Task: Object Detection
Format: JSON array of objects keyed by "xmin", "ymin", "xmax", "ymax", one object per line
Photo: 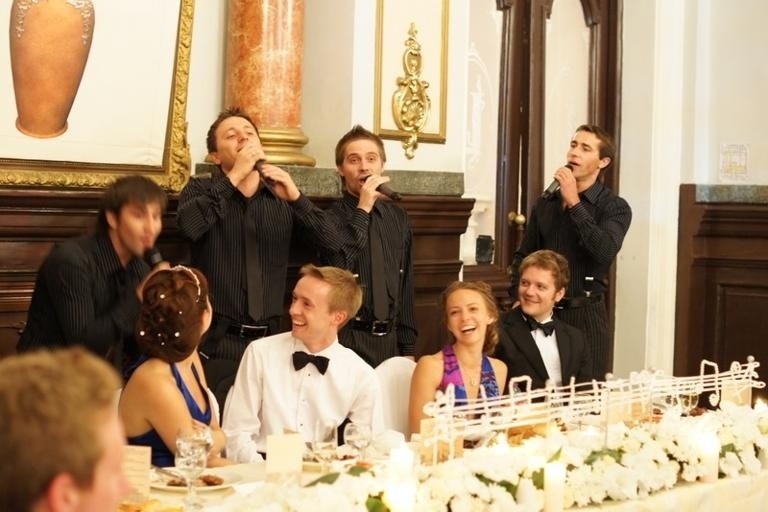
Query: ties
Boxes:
[
  {"xmin": 241, "ymin": 197, "xmax": 267, "ymax": 321},
  {"xmin": 367, "ymin": 212, "xmax": 391, "ymax": 321}
]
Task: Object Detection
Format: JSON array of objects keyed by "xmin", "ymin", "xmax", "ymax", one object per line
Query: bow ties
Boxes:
[
  {"xmin": 293, "ymin": 351, "xmax": 330, "ymax": 374},
  {"xmin": 527, "ymin": 319, "xmax": 556, "ymax": 337}
]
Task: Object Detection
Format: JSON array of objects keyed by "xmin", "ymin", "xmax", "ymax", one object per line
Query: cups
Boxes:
[{"xmin": 263, "ymin": 432, "xmax": 298, "ymax": 486}]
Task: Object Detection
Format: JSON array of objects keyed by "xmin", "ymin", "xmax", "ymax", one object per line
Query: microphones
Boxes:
[
  {"xmin": 255, "ymin": 158, "xmax": 276, "ymax": 188},
  {"xmin": 365, "ymin": 174, "xmax": 402, "ymax": 201},
  {"xmin": 142, "ymin": 246, "xmax": 162, "ymax": 270},
  {"xmin": 543, "ymin": 164, "xmax": 573, "ymax": 199}
]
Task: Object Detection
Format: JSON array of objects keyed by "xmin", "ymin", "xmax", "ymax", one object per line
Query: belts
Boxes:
[
  {"xmin": 553, "ymin": 296, "xmax": 601, "ymax": 309},
  {"xmin": 349, "ymin": 320, "xmax": 391, "ymax": 336},
  {"xmin": 229, "ymin": 324, "xmax": 268, "ymax": 341}
]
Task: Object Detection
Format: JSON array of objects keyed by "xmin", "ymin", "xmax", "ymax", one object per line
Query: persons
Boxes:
[
  {"xmin": 223, "ymin": 263, "xmax": 388, "ymax": 464},
  {"xmin": 409, "ymin": 279, "xmax": 508, "ymax": 434},
  {"xmin": 0, "ymin": 346, "xmax": 134, "ymax": 512},
  {"xmin": 16, "ymin": 175, "xmax": 163, "ymax": 361},
  {"xmin": 174, "ymin": 104, "xmax": 343, "ymax": 412},
  {"xmin": 322, "ymin": 124, "xmax": 418, "ymax": 368},
  {"xmin": 118, "ymin": 264, "xmax": 235, "ymax": 468},
  {"xmin": 513, "ymin": 124, "xmax": 631, "ymax": 383},
  {"xmin": 493, "ymin": 250, "xmax": 596, "ymax": 403}
]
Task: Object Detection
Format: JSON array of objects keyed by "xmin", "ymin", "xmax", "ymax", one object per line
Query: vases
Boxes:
[{"xmin": 9, "ymin": 0, "xmax": 95, "ymax": 139}]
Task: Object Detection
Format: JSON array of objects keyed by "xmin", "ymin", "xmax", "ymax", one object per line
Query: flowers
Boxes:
[{"xmin": 279, "ymin": 401, "xmax": 767, "ymax": 512}]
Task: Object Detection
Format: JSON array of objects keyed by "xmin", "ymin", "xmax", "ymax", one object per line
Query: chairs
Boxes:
[{"xmin": 368, "ymin": 354, "xmax": 417, "ymax": 442}]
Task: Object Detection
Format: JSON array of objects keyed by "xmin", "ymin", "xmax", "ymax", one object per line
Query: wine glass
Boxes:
[
  {"xmin": 311, "ymin": 417, "xmax": 339, "ymax": 477},
  {"xmin": 344, "ymin": 422, "xmax": 375, "ymax": 464},
  {"xmin": 172, "ymin": 422, "xmax": 213, "ymax": 512}
]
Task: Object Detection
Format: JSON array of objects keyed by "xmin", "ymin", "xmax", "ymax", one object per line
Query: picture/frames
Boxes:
[{"xmin": 0, "ymin": 0, "xmax": 194, "ymax": 199}]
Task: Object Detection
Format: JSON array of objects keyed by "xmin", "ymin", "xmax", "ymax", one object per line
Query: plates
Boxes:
[
  {"xmin": 299, "ymin": 444, "xmax": 356, "ymax": 472},
  {"xmin": 150, "ymin": 465, "xmax": 242, "ymax": 492}
]
